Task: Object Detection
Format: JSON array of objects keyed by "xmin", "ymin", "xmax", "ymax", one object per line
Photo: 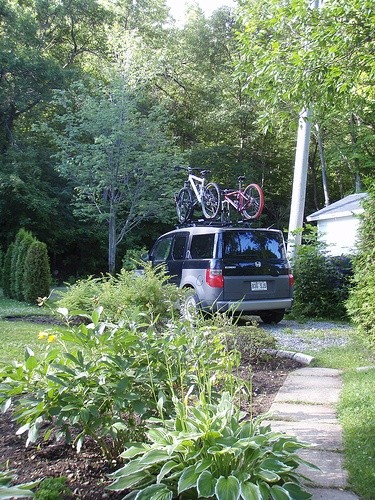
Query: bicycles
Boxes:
[{"xmin": 174, "ymin": 166, "xmax": 264, "ymax": 224}]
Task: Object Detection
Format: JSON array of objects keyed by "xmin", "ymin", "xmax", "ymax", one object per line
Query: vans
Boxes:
[{"xmin": 142, "ymin": 227, "xmax": 294, "ymax": 323}]
